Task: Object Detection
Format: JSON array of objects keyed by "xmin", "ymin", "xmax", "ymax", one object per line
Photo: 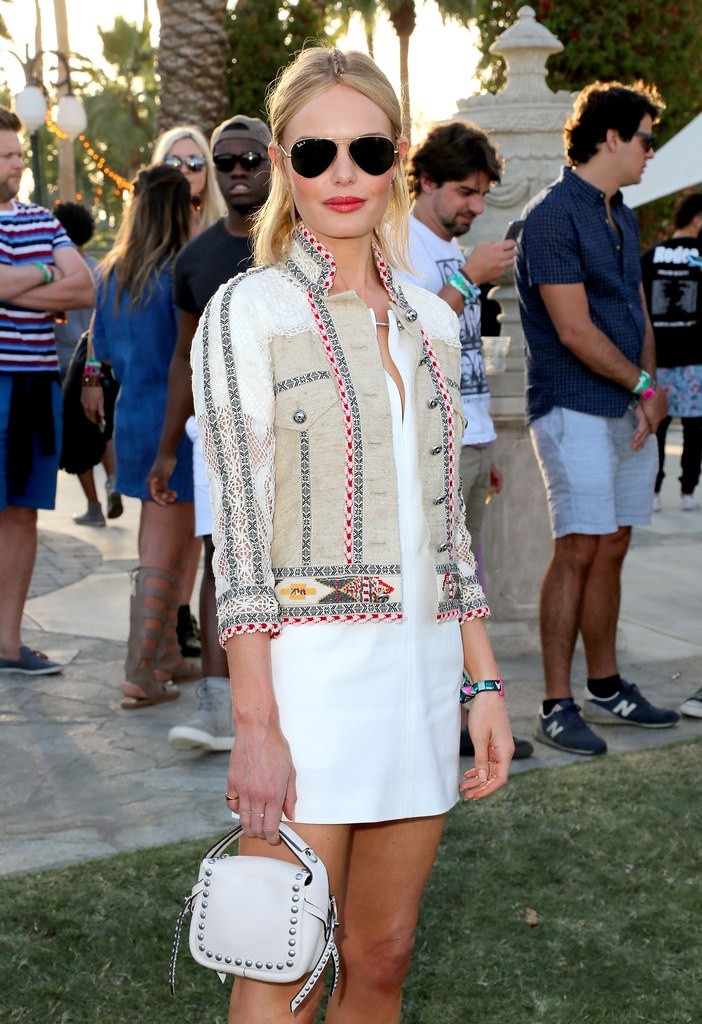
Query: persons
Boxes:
[
  {"xmin": 384, "ymin": 121, "xmax": 534, "ymax": 758},
  {"xmin": 0, "ymin": 109, "xmax": 97, "ymax": 675},
  {"xmin": 189, "ymin": 48, "xmax": 515, "ymax": 1024},
  {"xmin": 91, "ymin": 163, "xmax": 202, "ymax": 708},
  {"xmin": 640, "ymin": 192, "xmax": 702, "ymax": 511},
  {"xmin": 52, "ymin": 202, "xmax": 124, "ymax": 527},
  {"xmin": 81, "ymin": 127, "xmax": 229, "ymax": 657},
  {"xmin": 513, "ymin": 82, "xmax": 681, "ymax": 754},
  {"xmin": 147, "ymin": 115, "xmax": 272, "ymax": 751}
]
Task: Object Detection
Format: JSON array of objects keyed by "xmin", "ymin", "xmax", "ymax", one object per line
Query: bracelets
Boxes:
[
  {"xmin": 33, "ymin": 262, "xmax": 54, "ymax": 282},
  {"xmin": 628, "ymin": 395, "xmax": 639, "ymax": 410},
  {"xmin": 447, "ymin": 269, "xmax": 481, "ymax": 305},
  {"xmin": 460, "ymin": 674, "xmax": 504, "ymax": 704},
  {"xmin": 632, "ymin": 370, "xmax": 656, "ymax": 400},
  {"xmin": 82, "ymin": 358, "xmax": 104, "ymax": 387}
]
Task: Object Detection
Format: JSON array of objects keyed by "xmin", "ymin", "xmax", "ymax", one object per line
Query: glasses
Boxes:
[
  {"xmin": 213, "ymin": 152, "xmax": 267, "ymax": 172},
  {"xmin": 278, "ymin": 136, "xmax": 399, "ymax": 176},
  {"xmin": 636, "ymin": 132, "xmax": 656, "ymax": 152},
  {"xmin": 165, "ymin": 155, "xmax": 206, "ymax": 171}
]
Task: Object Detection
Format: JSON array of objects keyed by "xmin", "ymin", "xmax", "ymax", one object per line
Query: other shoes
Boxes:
[
  {"xmin": 73, "ymin": 503, "xmax": 105, "ymax": 526},
  {"xmin": 652, "ymin": 495, "xmax": 661, "ymax": 512},
  {"xmin": 105, "ymin": 475, "xmax": 124, "ymax": 518},
  {"xmin": 0, "ymin": 646, "xmax": 64, "ymax": 674},
  {"xmin": 682, "ymin": 494, "xmax": 693, "ymax": 511},
  {"xmin": 462, "ymin": 727, "xmax": 533, "ymax": 759},
  {"xmin": 680, "ymin": 687, "xmax": 702, "ymax": 718}
]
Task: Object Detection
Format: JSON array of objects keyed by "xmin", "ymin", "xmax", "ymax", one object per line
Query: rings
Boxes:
[
  {"xmin": 248, "ymin": 810, "xmax": 264, "ymax": 818},
  {"xmin": 225, "ymin": 793, "xmax": 239, "ymax": 800}
]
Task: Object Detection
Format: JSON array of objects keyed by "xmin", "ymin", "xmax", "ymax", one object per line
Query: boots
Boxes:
[
  {"xmin": 154, "ymin": 561, "xmax": 202, "ymax": 681},
  {"xmin": 177, "ymin": 604, "xmax": 204, "ymax": 671},
  {"xmin": 166, "ymin": 677, "xmax": 233, "ymax": 753},
  {"xmin": 120, "ymin": 566, "xmax": 180, "ymax": 708}
]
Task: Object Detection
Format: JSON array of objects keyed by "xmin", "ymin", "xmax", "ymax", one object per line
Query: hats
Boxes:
[{"xmin": 210, "ymin": 114, "xmax": 271, "ymax": 154}]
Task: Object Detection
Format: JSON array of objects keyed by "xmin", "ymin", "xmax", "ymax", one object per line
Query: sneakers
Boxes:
[
  {"xmin": 583, "ymin": 681, "xmax": 679, "ymax": 728},
  {"xmin": 534, "ymin": 700, "xmax": 606, "ymax": 753}
]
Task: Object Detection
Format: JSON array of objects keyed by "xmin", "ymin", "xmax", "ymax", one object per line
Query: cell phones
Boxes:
[
  {"xmin": 503, "ymin": 221, "xmax": 525, "ymax": 241},
  {"xmin": 96, "ymin": 411, "xmax": 106, "ymax": 433}
]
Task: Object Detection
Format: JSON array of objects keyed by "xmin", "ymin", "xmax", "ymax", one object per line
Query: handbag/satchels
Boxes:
[{"xmin": 170, "ymin": 821, "xmax": 340, "ymax": 1011}]
